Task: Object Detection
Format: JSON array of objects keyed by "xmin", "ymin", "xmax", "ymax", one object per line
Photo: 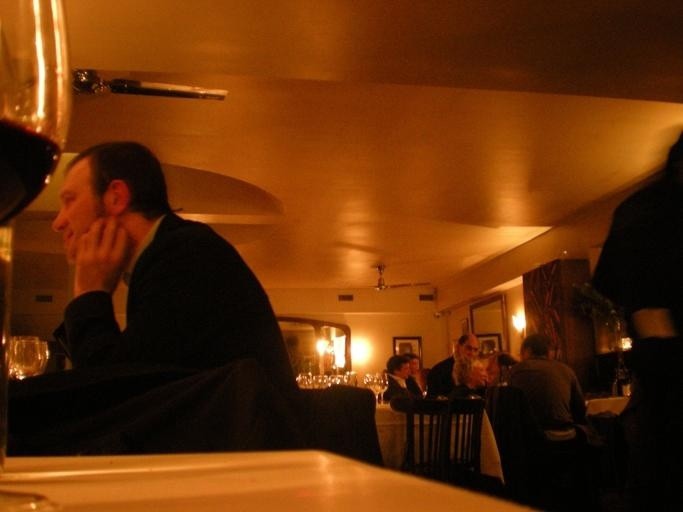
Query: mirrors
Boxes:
[{"xmin": 274, "ymin": 317, "xmax": 352, "ymax": 377}]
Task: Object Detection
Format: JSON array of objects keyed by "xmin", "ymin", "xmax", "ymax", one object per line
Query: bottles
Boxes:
[{"xmin": 609, "ymin": 360, "xmax": 631, "ymax": 396}]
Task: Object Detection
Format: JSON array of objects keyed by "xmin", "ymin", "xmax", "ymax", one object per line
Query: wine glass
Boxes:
[{"xmin": 295, "ymin": 371, "xmax": 387, "ymax": 407}]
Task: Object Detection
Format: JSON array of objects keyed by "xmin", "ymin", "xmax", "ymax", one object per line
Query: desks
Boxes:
[{"xmin": 0, "ymin": 449, "xmax": 545, "ymax": 512}]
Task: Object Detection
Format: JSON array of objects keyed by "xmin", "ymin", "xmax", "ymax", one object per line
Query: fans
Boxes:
[{"xmin": 334, "ymin": 260, "xmax": 431, "ymax": 292}]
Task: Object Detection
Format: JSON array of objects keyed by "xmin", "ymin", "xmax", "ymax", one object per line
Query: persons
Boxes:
[
  {"xmin": 585, "ymin": 128, "xmax": 683, "ymax": 509},
  {"xmin": 504, "ymin": 333, "xmax": 593, "ymax": 447},
  {"xmin": 402, "ymin": 351, "xmax": 425, "ymax": 400},
  {"xmin": 478, "ymin": 338, "xmax": 496, "ymax": 360},
  {"xmin": 0, "ymin": 137, "xmax": 317, "ymax": 455},
  {"xmin": 490, "ymin": 353, "xmax": 517, "ymax": 385},
  {"xmin": 445, "ymin": 359, "xmax": 487, "ymax": 402},
  {"xmin": 377, "ymin": 355, "xmax": 416, "ymax": 405},
  {"xmin": 425, "ymin": 332, "xmax": 488, "ymax": 397}
]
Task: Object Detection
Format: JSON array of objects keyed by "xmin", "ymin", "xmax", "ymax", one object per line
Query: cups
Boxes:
[
  {"xmin": 0, "ymin": 0, "xmax": 69, "ymax": 225},
  {"xmin": 4, "ymin": 335, "xmax": 49, "ymax": 380}
]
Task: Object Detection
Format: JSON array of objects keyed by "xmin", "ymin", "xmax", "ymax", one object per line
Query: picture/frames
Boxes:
[
  {"xmin": 392, "ymin": 336, "xmax": 422, "ymax": 359},
  {"xmin": 474, "ymin": 333, "xmax": 501, "ymax": 359},
  {"xmin": 467, "ymin": 293, "xmax": 510, "ymax": 355}
]
